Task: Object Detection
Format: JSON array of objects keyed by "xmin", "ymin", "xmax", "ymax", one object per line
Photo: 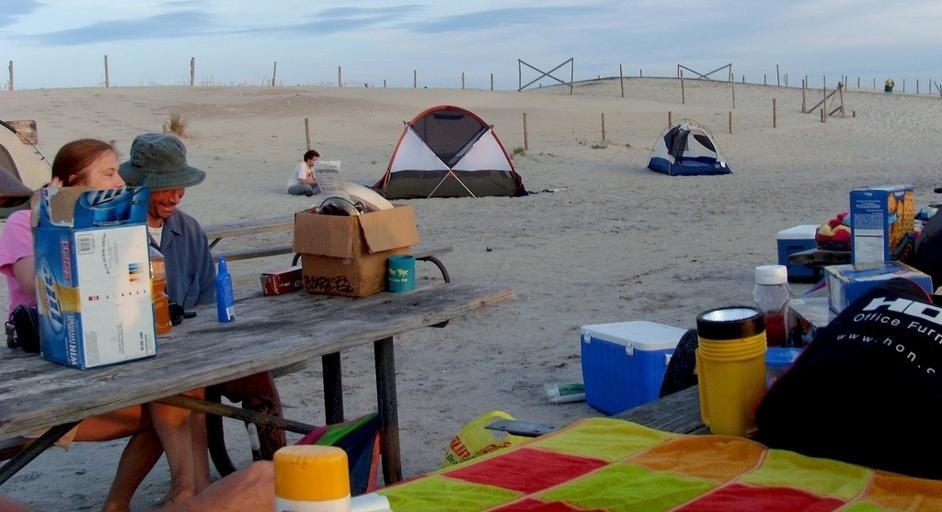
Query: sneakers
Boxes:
[{"xmin": 301, "ymin": 191, "xmax": 313, "ymax": 196}]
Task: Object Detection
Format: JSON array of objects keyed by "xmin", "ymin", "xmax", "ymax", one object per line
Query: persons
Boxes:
[
  {"xmin": 287, "ymin": 148, "xmax": 322, "ymax": 196},
  {"xmin": 0, "ymin": 132, "xmax": 288, "ymax": 512}
]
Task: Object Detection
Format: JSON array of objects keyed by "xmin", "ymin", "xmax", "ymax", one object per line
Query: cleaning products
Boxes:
[{"xmin": 217, "ymin": 256, "xmax": 236, "ymax": 322}]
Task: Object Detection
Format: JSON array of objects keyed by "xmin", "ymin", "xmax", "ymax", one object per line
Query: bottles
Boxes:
[
  {"xmin": 753, "ymin": 265, "xmax": 792, "ymax": 347},
  {"xmin": 214, "ymin": 253, "xmax": 234, "ymax": 321},
  {"xmin": 272, "ymin": 444, "xmax": 353, "ymax": 512}
]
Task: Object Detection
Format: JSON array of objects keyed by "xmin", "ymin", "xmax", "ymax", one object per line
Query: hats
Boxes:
[{"xmin": 118, "ymin": 133, "xmax": 206, "ymax": 191}]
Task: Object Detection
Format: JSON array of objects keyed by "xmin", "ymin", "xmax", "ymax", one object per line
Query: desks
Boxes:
[
  {"xmin": 360, "ymin": 365, "xmax": 942, "ymax": 512},
  {"xmin": 0, "ymin": 268, "xmax": 513, "ymax": 512}
]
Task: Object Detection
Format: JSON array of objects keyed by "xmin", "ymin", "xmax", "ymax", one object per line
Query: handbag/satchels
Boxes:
[{"xmin": 13, "ymin": 303, "xmax": 40, "ymax": 353}]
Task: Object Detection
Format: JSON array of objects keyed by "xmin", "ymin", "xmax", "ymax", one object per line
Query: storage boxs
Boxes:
[
  {"xmin": 292, "ymin": 201, "xmax": 420, "ymax": 299},
  {"xmin": 849, "ymin": 183, "xmax": 914, "ymax": 265},
  {"xmin": 581, "ymin": 320, "xmax": 688, "ymax": 418},
  {"xmin": 823, "ymin": 260, "xmax": 933, "ymax": 316},
  {"xmin": 777, "ymin": 223, "xmax": 828, "ymax": 284},
  {"xmin": 27, "ymin": 185, "xmax": 157, "ymax": 369}
]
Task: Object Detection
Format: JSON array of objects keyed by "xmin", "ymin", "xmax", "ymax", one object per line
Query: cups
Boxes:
[{"xmin": 386, "ymin": 254, "xmax": 414, "ymax": 294}]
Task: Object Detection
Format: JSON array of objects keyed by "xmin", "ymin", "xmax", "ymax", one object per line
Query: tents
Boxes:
[
  {"xmin": 375, "ymin": 105, "xmax": 528, "ymax": 201},
  {"xmin": 647, "ymin": 121, "xmax": 733, "ymax": 177},
  {"xmin": 0, "ymin": 119, "xmax": 57, "ymax": 215}
]
